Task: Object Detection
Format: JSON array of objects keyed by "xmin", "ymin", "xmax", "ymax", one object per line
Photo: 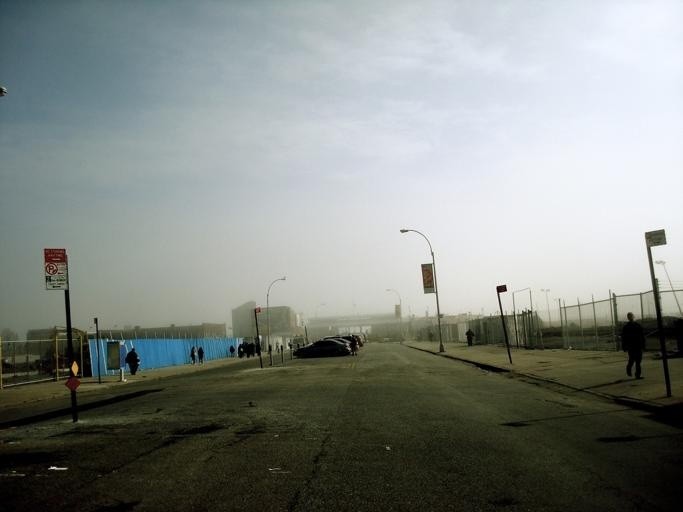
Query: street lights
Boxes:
[
  {"xmin": 540, "ymin": 288, "xmax": 552, "ymax": 328},
  {"xmin": 400, "ymin": 229, "xmax": 445, "ymax": 352},
  {"xmin": 385, "ymin": 288, "xmax": 403, "ymax": 345},
  {"xmin": 267, "ymin": 276, "xmax": 286, "ymax": 366},
  {"xmin": 655, "ymin": 260, "xmax": 682, "ymax": 316}
]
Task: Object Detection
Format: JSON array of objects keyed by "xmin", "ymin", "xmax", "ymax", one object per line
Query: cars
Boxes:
[{"xmin": 293, "ymin": 333, "xmax": 369, "ymax": 358}]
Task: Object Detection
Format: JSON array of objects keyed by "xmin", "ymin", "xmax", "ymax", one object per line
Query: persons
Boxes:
[
  {"xmin": 198, "ymin": 347, "xmax": 204, "ymax": 362},
  {"xmin": 191, "ymin": 347, "xmax": 197, "ymax": 363},
  {"xmin": 126, "ymin": 348, "xmax": 139, "ymax": 375},
  {"xmin": 621, "ymin": 312, "xmax": 647, "ymax": 378},
  {"xmin": 465, "ymin": 329, "xmax": 474, "ymax": 346},
  {"xmin": 237, "ymin": 343, "xmax": 260, "ymax": 359},
  {"xmin": 351, "ymin": 335, "xmax": 358, "ymax": 356},
  {"xmin": 230, "ymin": 346, "xmax": 235, "ymax": 358}
]
